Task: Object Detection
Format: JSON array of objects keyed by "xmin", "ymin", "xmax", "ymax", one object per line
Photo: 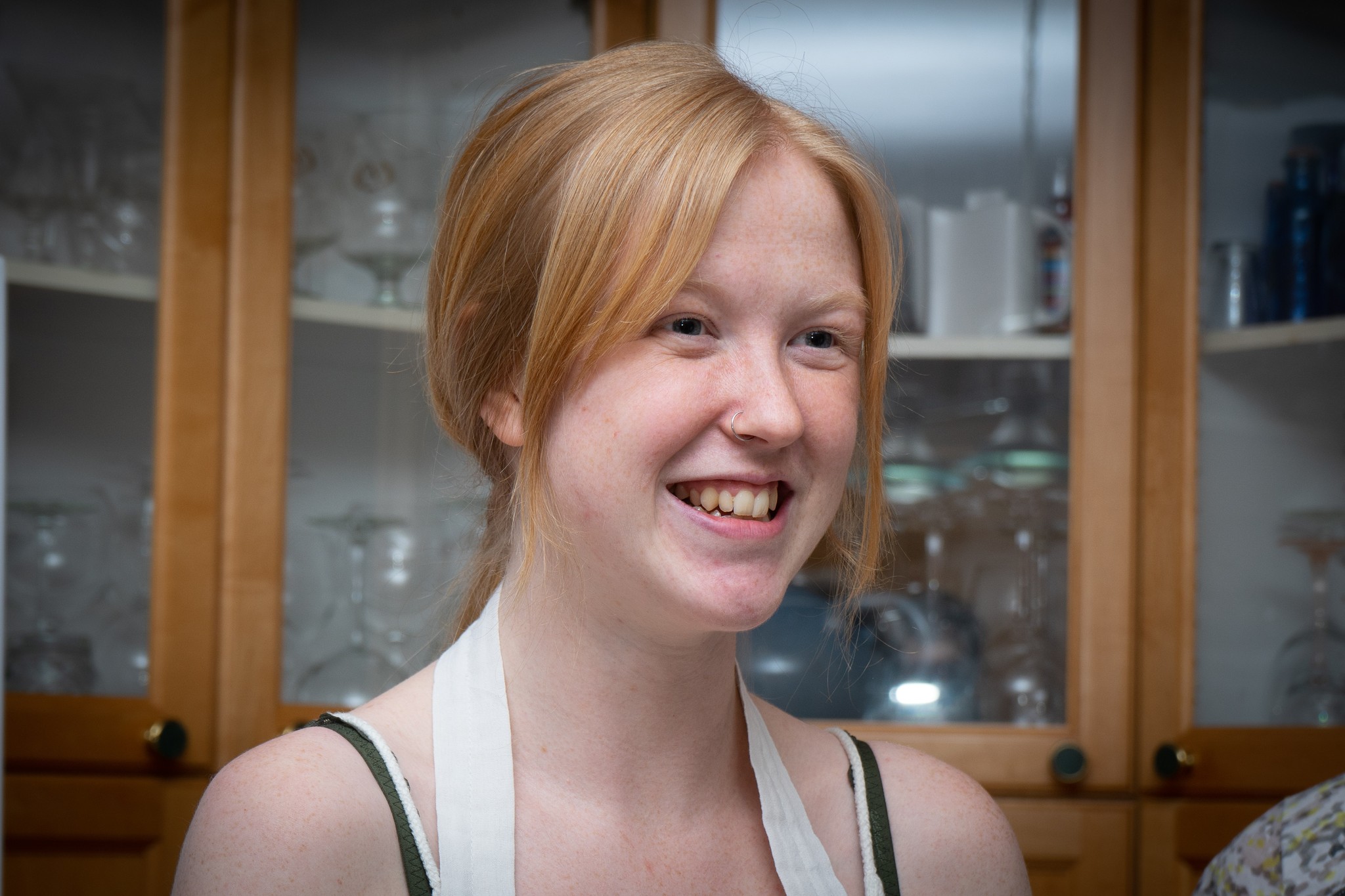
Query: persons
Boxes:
[{"xmin": 169, "ymin": 39, "xmax": 1032, "ymax": 896}]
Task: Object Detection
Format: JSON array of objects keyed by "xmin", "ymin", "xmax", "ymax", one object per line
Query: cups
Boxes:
[
  {"xmin": 928, "ymin": 206, "xmax": 1073, "ymax": 341},
  {"xmin": 1208, "ymin": 244, "xmax": 1259, "ymax": 329}
]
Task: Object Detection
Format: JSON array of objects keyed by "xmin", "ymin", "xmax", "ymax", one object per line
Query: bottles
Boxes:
[
  {"xmin": 845, "ymin": 369, "xmax": 1064, "ymax": 735},
  {"xmin": 1257, "ymin": 156, "xmax": 1328, "ymax": 323}
]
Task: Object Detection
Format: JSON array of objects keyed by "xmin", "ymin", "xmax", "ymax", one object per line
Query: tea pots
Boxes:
[{"xmin": 739, "ymin": 570, "xmax": 937, "ymax": 718}]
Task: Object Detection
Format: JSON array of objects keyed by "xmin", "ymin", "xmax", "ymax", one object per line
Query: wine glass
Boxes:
[
  {"xmin": 1279, "ymin": 511, "xmax": 1344, "ymax": 725},
  {"xmin": 3, "ymin": 496, "xmax": 152, "ymax": 700},
  {"xmin": 0, "ymin": 121, "xmax": 439, "ymax": 313},
  {"xmin": 287, "ymin": 507, "xmax": 487, "ymax": 707}
]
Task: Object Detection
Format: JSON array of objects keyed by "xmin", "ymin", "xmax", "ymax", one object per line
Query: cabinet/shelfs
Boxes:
[{"xmin": 1, "ymin": 1, "xmax": 1345, "ymax": 896}]
